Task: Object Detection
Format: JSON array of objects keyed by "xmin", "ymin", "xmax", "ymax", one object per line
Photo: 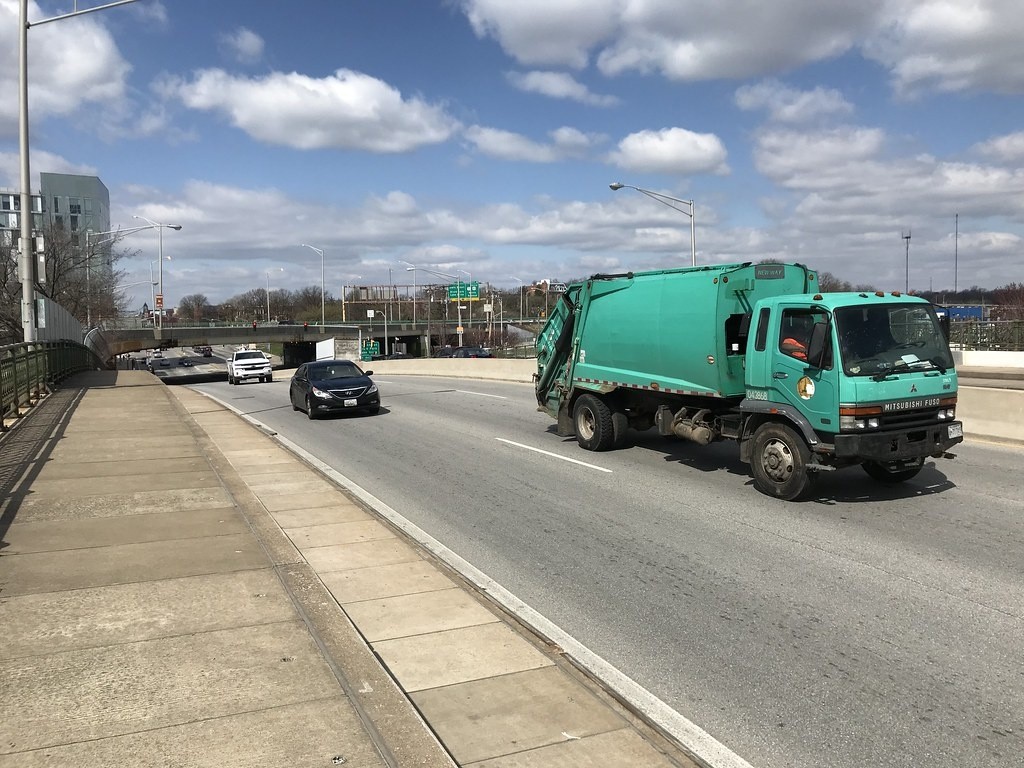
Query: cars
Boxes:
[
  {"xmin": 192, "ymin": 345, "xmax": 213, "ymax": 358},
  {"xmin": 160, "ymin": 358, "xmax": 170, "ymax": 366},
  {"xmin": 153, "ymin": 347, "xmax": 167, "ymax": 358},
  {"xmin": 372, "ymin": 345, "xmax": 496, "ymax": 361},
  {"xmin": 179, "ymin": 357, "xmax": 193, "ymax": 367},
  {"xmin": 290, "ymin": 358, "xmax": 380, "ymax": 420}
]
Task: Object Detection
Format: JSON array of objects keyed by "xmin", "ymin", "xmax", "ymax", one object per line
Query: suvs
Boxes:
[{"xmin": 226, "ymin": 348, "xmax": 273, "ymax": 386}]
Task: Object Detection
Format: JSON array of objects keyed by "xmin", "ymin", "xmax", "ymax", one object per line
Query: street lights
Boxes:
[
  {"xmin": 267, "ymin": 267, "xmax": 284, "ymax": 323},
  {"xmin": 151, "ymin": 255, "xmax": 172, "ymax": 329},
  {"xmin": 457, "ymin": 269, "xmax": 471, "ymax": 322},
  {"xmin": 376, "ymin": 310, "xmax": 388, "ymax": 356},
  {"xmin": 302, "ymin": 244, "xmax": 325, "ymax": 325},
  {"xmin": 132, "ymin": 215, "xmax": 163, "ymax": 329},
  {"xmin": 87, "ymin": 225, "xmax": 182, "ymax": 338},
  {"xmin": 609, "ymin": 182, "xmax": 696, "ymax": 267},
  {"xmin": 511, "ymin": 276, "xmax": 523, "ymax": 321},
  {"xmin": 408, "ymin": 266, "xmax": 462, "ymax": 347}
]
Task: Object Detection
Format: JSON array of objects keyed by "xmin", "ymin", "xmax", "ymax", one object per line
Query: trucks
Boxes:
[{"xmin": 532, "ymin": 261, "xmax": 965, "ymax": 502}]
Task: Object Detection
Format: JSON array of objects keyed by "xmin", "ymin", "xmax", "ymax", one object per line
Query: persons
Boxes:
[
  {"xmin": 782, "ymin": 317, "xmax": 815, "ymax": 358},
  {"xmin": 855, "ymin": 309, "xmax": 906, "ymax": 357}
]
